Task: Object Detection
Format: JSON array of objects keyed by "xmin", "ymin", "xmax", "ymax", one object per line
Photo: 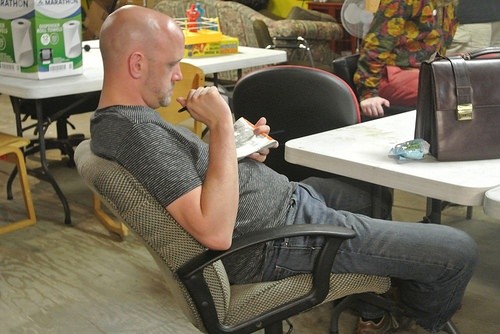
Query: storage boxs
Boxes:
[
  {"xmin": 0, "ymin": 0, "xmax": 83, "ymax": 80},
  {"xmin": 82, "ymin": 0, "xmax": 115, "ymax": 37}
]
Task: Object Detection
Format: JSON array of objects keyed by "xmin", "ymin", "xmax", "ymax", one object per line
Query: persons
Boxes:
[
  {"xmin": 353, "ymin": 0, "xmax": 465, "ymax": 118},
  {"xmin": 90, "ymin": 5, "xmax": 478, "ymax": 334}
]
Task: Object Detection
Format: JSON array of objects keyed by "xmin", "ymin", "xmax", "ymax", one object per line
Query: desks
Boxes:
[{"xmin": 0, "ymin": 40, "xmax": 500, "ymax": 225}]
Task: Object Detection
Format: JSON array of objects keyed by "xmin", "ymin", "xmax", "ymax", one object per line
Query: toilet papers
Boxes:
[
  {"xmin": 10, "ymin": 18, "xmax": 34, "ymax": 67},
  {"xmin": 62, "ymin": 20, "xmax": 82, "ymax": 59}
]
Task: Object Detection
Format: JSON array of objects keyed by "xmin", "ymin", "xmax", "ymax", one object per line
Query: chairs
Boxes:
[
  {"xmin": 92, "ymin": 62, "xmax": 204, "ymax": 238},
  {"xmin": 73, "ymin": 140, "xmax": 390, "ymax": 334},
  {"xmin": 252, "ymin": 19, "xmax": 315, "ymax": 69},
  {"xmin": 0, "ymin": 131, "xmax": 36, "ymax": 237},
  {"xmin": 232, "ymin": 65, "xmax": 360, "ymax": 182},
  {"xmin": 330, "ymin": 53, "xmax": 415, "ymax": 122}
]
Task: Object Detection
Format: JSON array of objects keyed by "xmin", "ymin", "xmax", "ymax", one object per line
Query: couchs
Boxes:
[{"xmin": 152, "ymin": 0, "xmax": 342, "ymax": 98}]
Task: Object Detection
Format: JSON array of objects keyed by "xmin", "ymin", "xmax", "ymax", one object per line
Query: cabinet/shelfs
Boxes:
[{"xmin": 307, "ymin": 2, "xmax": 357, "ymax": 54}]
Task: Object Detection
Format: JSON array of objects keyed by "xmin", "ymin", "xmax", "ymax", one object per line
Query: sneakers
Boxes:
[{"xmin": 356, "ymin": 316, "xmax": 387, "ymax": 334}]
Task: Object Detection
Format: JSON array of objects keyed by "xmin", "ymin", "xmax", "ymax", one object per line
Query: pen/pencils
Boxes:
[{"xmin": 178, "ymin": 106, "xmax": 187, "ymax": 113}]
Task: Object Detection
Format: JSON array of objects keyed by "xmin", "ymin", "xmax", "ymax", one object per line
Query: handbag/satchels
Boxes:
[{"xmin": 414, "ymin": 47, "xmax": 500, "ymax": 161}]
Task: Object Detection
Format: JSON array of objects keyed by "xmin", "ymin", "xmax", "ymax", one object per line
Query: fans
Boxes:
[{"xmin": 340, "ymin": 0, "xmax": 381, "ymax": 53}]
